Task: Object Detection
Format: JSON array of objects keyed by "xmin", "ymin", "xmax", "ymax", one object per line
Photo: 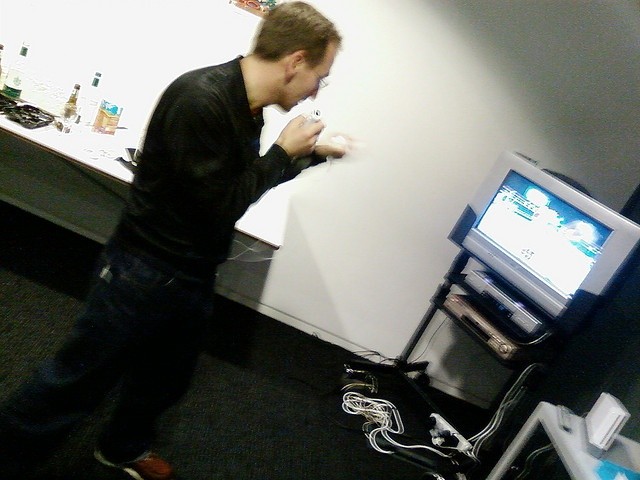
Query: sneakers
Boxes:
[{"xmin": 94, "ymin": 445, "xmax": 174, "ymax": 480}]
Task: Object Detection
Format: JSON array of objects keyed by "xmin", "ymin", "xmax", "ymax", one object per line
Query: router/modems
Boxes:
[{"xmin": 585, "ymin": 392, "xmax": 631, "ymax": 453}]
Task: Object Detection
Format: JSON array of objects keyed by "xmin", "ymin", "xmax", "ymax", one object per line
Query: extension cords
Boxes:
[{"xmin": 430, "ymin": 412, "xmax": 473, "ymax": 453}]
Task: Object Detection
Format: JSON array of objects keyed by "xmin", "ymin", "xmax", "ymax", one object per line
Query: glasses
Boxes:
[{"xmin": 310, "ymin": 67, "xmax": 331, "ymax": 90}]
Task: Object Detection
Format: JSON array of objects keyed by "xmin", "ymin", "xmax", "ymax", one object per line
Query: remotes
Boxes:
[{"xmin": 558, "ymin": 404, "xmax": 571, "ymax": 431}]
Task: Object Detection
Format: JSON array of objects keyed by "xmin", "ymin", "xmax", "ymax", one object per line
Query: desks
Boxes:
[{"xmin": 479, "ymin": 401, "xmax": 640, "ymax": 480}]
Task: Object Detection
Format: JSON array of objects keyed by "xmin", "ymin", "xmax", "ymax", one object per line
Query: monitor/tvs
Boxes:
[{"xmin": 447, "ymin": 150, "xmax": 640, "ymax": 318}]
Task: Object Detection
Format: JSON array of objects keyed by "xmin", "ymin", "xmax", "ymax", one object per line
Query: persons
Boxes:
[{"xmin": 0, "ymin": 0, "xmax": 347, "ymax": 480}]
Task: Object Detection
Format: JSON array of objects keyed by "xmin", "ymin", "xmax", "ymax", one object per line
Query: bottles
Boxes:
[
  {"xmin": 82, "ymin": 71, "xmax": 102, "ymax": 126},
  {"xmin": 73, "ymin": 70, "xmax": 103, "ymax": 124},
  {"xmin": 56, "ymin": 83, "xmax": 82, "ymax": 137},
  {"xmin": 0, "ymin": 40, "xmax": 31, "ymax": 112}
]
